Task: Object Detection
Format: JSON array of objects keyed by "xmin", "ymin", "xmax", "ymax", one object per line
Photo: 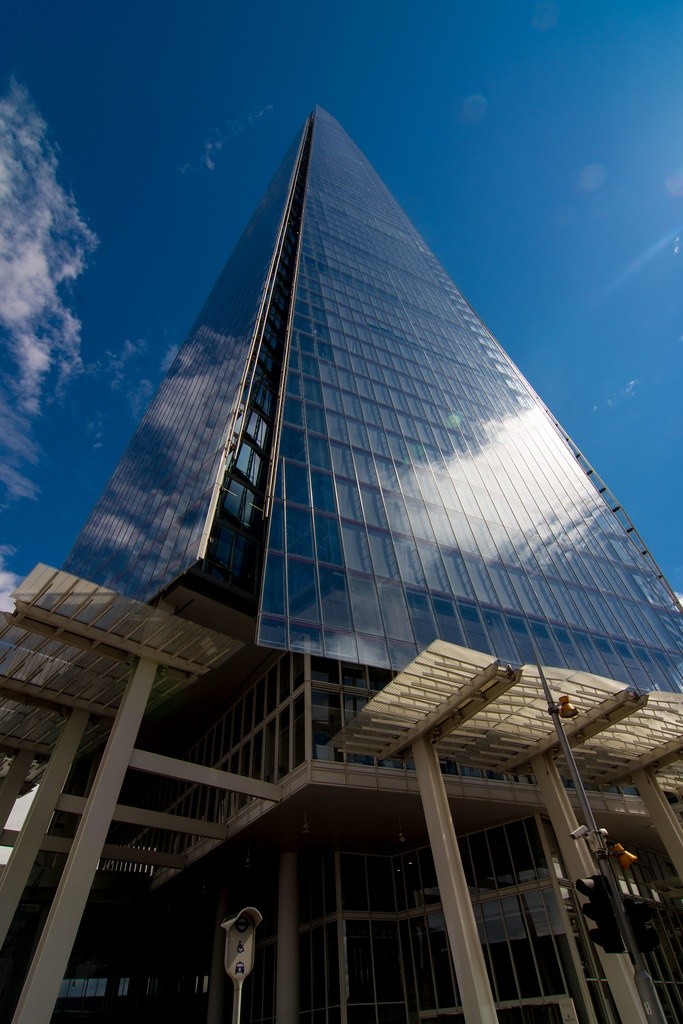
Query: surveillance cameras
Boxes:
[{"xmin": 570, "ymin": 825, "xmax": 588, "ymax": 840}]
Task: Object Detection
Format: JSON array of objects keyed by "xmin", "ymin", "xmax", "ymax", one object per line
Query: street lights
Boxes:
[{"xmin": 530, "ymin": 643, "xmax": 669, "ymax": 1024}]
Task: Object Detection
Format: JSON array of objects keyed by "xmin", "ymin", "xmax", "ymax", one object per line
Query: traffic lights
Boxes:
[{"xmin": 575, "ymin": 874, "xmax": 626, "ymax": 954}]
